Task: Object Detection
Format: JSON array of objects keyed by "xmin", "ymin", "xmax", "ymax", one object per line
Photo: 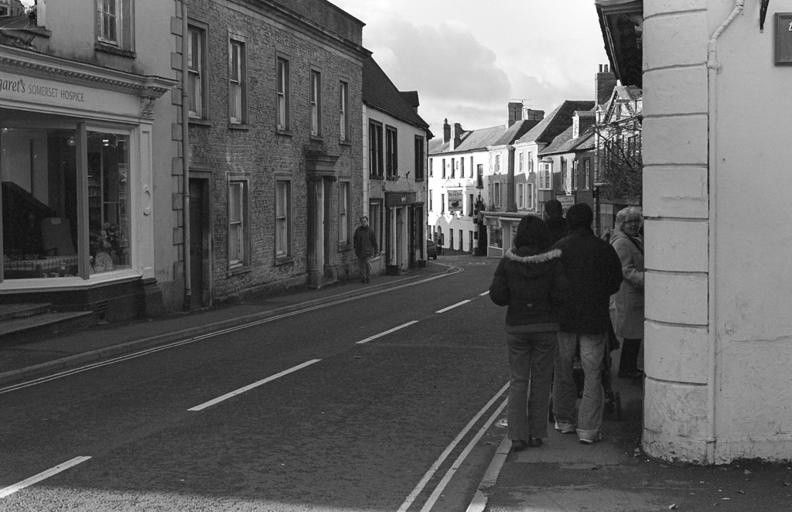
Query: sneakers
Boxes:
[
  {"xmin": 619, "ymin": 367, "xmax": 644, "ymax": 379},
  {"xmin": 510, "ymin": 434, "xmax": 542, "ymax": 452},
  {"xmin": 361, "ymin": 275, "xmax": 370, "ymax": 283},
  {"xmin": 553, "ymin": 419, "xmax": 606, "ymax": 444}
]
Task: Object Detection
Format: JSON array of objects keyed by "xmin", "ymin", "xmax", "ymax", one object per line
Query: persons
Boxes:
[
  {"xmin": 609, "ymin": 206, "xmax": 644, "ymax": 379},
  {"xmin": 489, "ymin": 199, "xmax": 623, "ymax": 450},
  {"xmin": 354, "ymin": 216, "xmax": 378, "ymax": 284}
]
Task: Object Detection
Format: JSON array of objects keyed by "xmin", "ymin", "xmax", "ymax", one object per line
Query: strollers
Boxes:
[{"xmin": 548, "ymin": 307, "xmax": 626, "ymax": 423}]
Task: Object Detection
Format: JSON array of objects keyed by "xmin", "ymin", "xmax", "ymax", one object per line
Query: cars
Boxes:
[{"xmin": 427, "ymin": 239, "xmax": 438, "ymax": 260}]
